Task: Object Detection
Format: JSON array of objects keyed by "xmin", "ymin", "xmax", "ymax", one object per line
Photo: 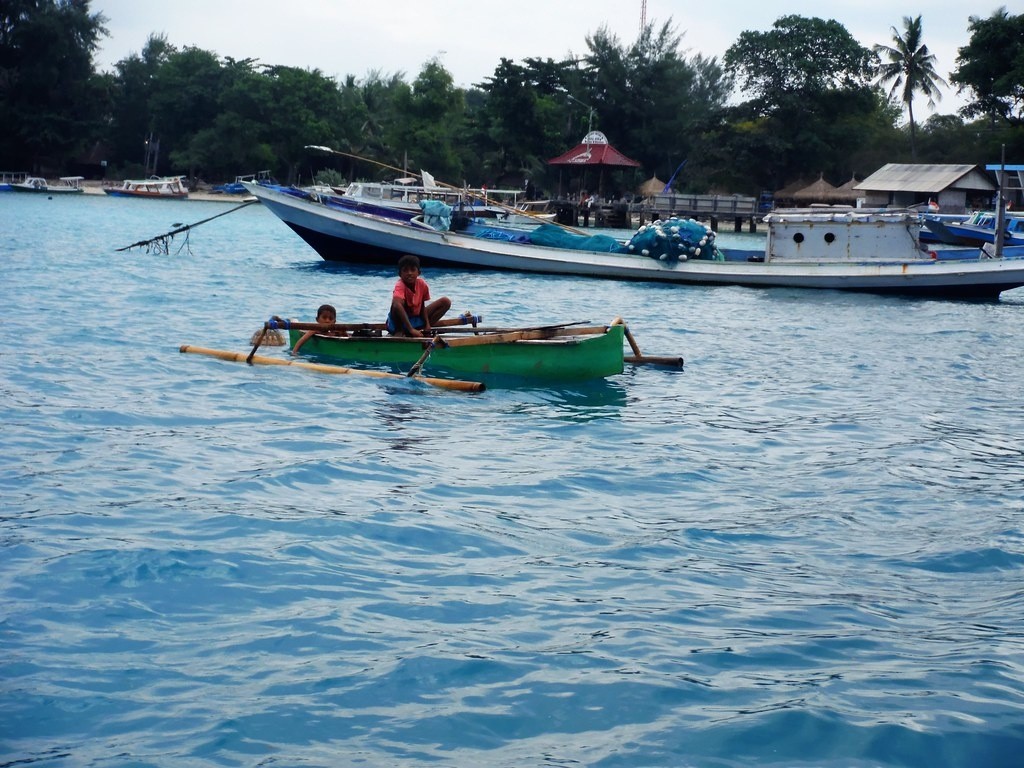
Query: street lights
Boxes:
[{"xmin": 566, "ymin": 93, "xmax": 594, "ymax": 144}]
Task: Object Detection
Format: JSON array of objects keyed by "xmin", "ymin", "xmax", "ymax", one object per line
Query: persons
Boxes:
[
  {"xmin": 293, "ymin": 305, "xmax": 351, "ymax": 354},
  {"xmin": 387, "ymin": 255, "xmax": 451, "ymax": 337}
]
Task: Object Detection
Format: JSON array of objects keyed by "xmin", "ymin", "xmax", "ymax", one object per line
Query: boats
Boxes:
[
  {"xmin": 286, "ymin": 320, "xmax": 624, "ymax": 380},
  {"xmin": 235, "ymin": 145, "xmax": 1024, "ymax": 296},
  {"xmin": 102, "ymin": 175, "xmax": 189, "ymax": 198},
  {"xmin": 0, "ymin": 171, "xmax": 85, "ymax": 193},
  {"xmin": 213, "ymin": 170, "xmax": 281, "ymax": 193}
]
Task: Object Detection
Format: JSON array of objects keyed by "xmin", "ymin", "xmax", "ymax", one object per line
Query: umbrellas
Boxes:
[
  {"xmin": 793, "ymin": 175, "xmax": 837, "ymax": 204},
  {"xmin": 774, "ymin": 176, "xmax": 808, "ymax": 208},
  {"xmin": 829, "ymin": 177, "xmax": 865, "ymax": 205}
]
